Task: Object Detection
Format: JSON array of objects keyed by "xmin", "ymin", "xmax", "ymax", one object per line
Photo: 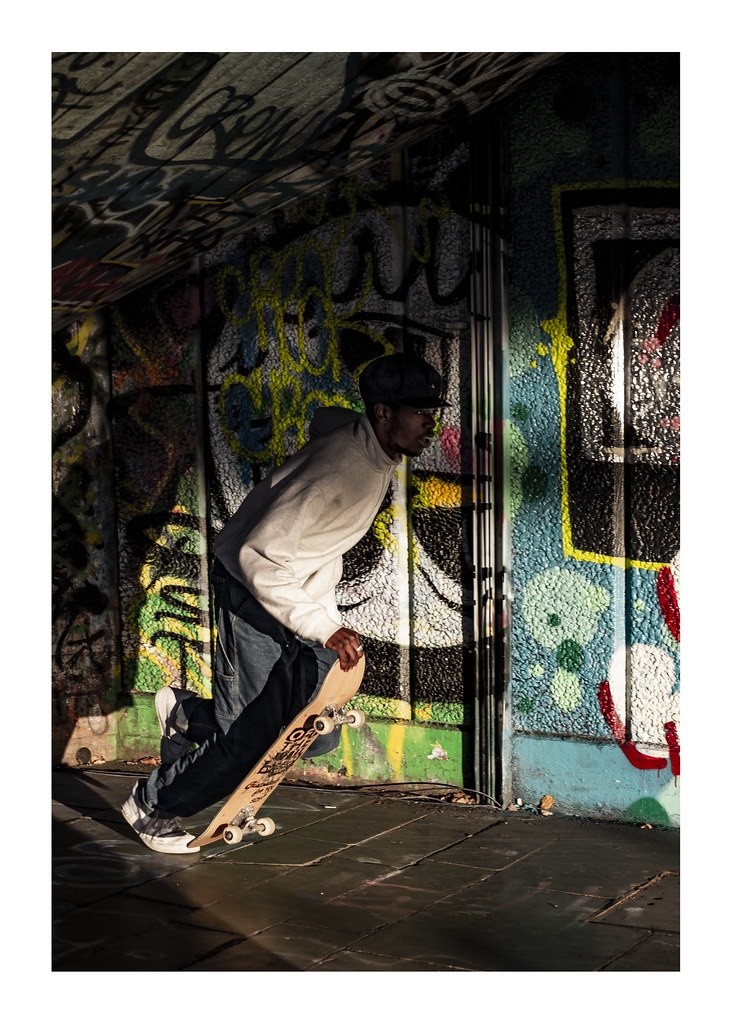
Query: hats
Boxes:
[{"xmin": 359, "ymin": 354, "xmax": 453, "ymax": 409}]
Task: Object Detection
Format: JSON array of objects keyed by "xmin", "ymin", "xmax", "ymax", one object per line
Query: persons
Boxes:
[{"xmin": 117, "ymin": 358, "xmax": 439, "ymax": 856}]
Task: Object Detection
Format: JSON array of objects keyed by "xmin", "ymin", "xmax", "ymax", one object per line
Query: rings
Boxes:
[{"xmin": 355, "ymin": 643, "xmax": 365, "ymax": 651}]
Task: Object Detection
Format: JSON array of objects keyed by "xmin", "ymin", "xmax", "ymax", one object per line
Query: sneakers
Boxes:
[
  {"xmin": 120, "ymin": 794, "xmax": 200, "ymax": 853},
  {"xmin": 155, "ymin": 685, "xmax": 198, "ymax": 739}
]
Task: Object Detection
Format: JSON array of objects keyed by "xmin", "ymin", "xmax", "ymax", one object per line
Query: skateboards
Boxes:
[{"xmin": 185, "ymin": 649, "xmax": 368, "ymax": 849}]
